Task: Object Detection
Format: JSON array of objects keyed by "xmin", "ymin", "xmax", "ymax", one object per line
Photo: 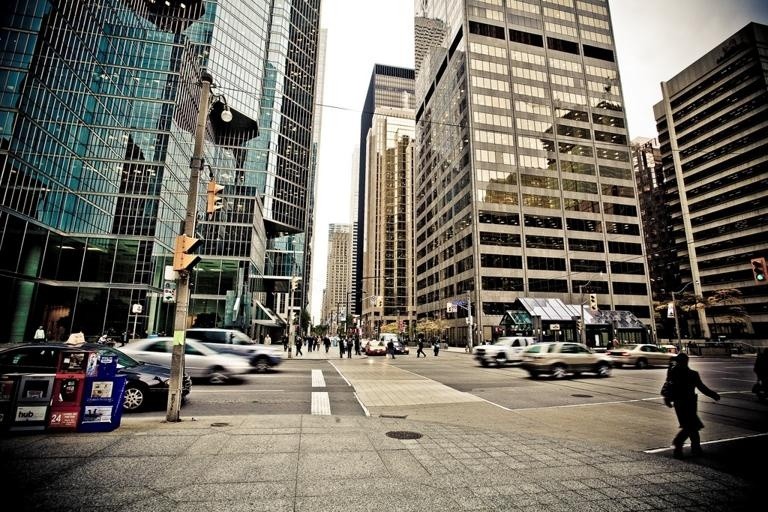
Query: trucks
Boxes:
[{"xmin": 378, "ymin": 332, "xmax": 398, "ymax": 348}]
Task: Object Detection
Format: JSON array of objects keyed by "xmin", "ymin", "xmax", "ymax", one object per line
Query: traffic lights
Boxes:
[
  {"xmin": 589, "ymin": 293, "xmax": 598, "ymax": 311},
  {"xmin": 290, "ymin": 277, "xmax": 299, "ymax": 291},
  {"xmin": 750, "ymin": 257, "xmax": 767, "ymax": 286},
  {"xmin": 171, "ymin": 233, "xmax": 202, "ymax": 275},
  {"xmin": 205, "ymin": 179, "xmax": 224, "ymax": 214}
]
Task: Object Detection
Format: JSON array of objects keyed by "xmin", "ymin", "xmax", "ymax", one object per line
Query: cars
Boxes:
[
  {"xmin": 332, "ymin": 335, "xmax": 376, "ymax": 352},
  {"xmin": 604, "ymin": 344, "xmax": 677, "ymax": 369},
  {"xmin": 364, "ymin": 339, "xmax": 386, "ymax": 357},
  {"xmin": 115, "ymin": 335, "xmax": 253, "ymax": 385},
  {"xmin": 389, "ymin": 341, "xmax": 409, "ymax": 355},
  {"xmin": 0, "ymin": 340, "xmax": 193, "ymax": 413}
]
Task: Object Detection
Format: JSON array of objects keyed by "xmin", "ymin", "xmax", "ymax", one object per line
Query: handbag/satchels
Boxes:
[
  {"xmin": 751, "ymin": 379, "xmax": 763, "ymax": 394},
  {"xmin": 660, "ymin": 379, "xmax": 674, "ymax": 403}
]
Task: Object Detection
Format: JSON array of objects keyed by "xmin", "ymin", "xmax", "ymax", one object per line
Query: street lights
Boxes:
[
  {"xmin": 321, "ymin": 309, "xmax": 342, "ymax": 335},
  {"xmin": 336, "ymin": 301, "xmax": 350, "ymax": 329},
  {"xmin": 578, "ymin": 270, "xmax": 603, "ymax": 346},
  {"xmin": 346, "ymin": 290, "xmax": 367, "ymax": 329},
  {"xmin": 360, "ymin": 276, "xmax": 390, "ymax": 280},
  {"xmin": 670, "ymin": 279, "xmax": 700, "ymax": 354},
  {"xmin": 161, "ymin": 72, "xmax": 233, "ymax": 424}
]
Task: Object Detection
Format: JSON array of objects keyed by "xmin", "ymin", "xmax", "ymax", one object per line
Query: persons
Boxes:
[
  {"xmin": 260, "ymin": 330, "xmax": 443, "ymax": 359},
  {"xmin": 613, "ymin": 337, "xmax": 619, "ymax": 350},
  {"xmin": 751, "ymin": 348, "xmax": 768, "ymax": 396},
  {"xmin": 33, "ymin": 325, "xmax": 46, "ymax": 342},
  {"xmin": 606, "ymin": 341, "xmax": 612, "ymax": 350},
  {"xmin": 660, "ymin": 353, "xmax": 720, "ymax": 458}
]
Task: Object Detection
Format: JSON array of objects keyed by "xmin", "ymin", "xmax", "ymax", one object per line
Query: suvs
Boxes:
[
  {"xmin": 520, "ymin": 342, "xmax": 613, "ymax": 381},
  {"xmin": 184, "ymin": 328, "xmax": 289, "ymax": 374},
  {"xmin": 471, "ymin": 337, "xmax": 537, "ymax": 367}
]
